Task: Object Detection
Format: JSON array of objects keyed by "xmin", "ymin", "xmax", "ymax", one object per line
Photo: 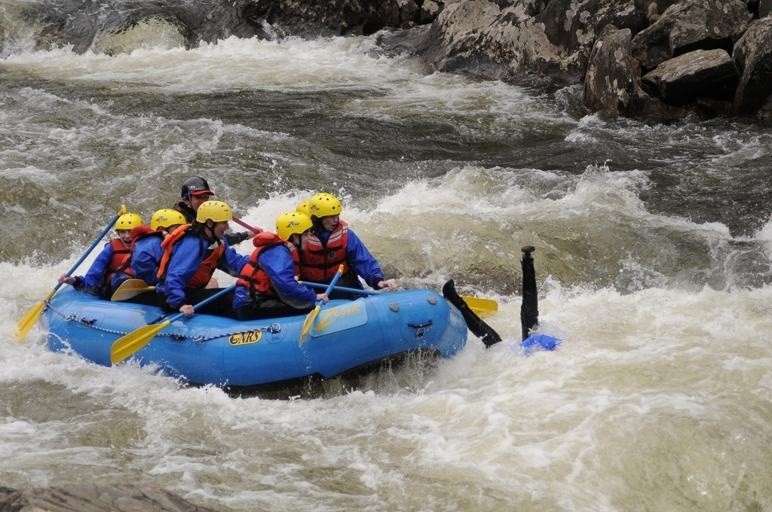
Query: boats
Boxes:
[{"xmin": 42, "ymin": 271, "xmax": 468, "ymax": 401}]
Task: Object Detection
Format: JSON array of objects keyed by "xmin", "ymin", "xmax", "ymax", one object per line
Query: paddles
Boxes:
[
  {"xmin": 110, "ymin": 283, "xmax": 236, "ymax": 365},
  {"xmin": 300, "ymin": 261, "xmax": 345, "ymax": 350},
  {"xmin": 10, "ymin": 205, "xmax": 127, "ymax": 342},
  {"xmin": 295, "ymin": 280, "xmax": 499, "ymax": 315},
  {"xmin": 111, "ymin": 276, "xmax": 159, "ymax": 302}
]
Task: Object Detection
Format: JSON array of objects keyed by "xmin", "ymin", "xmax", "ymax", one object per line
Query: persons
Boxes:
[
  {"xmin": 155, "ymin": 200, "xmax": 253, "ymax": 316},
  {"xmin": 231, "ymin": 211, "xmax": 329, "ymax": 316},
  {"xmin": 295, "ymin": 175, "xmax": 398, "ymax": 301},
  {"xmin": 104, "ymin": 207, "xmax": 186, "ymax": 308},
  {"xmin": 174, "ymin": 177, "xmax": 265, "ymax": 245},
  {"xmin": 62, "ymin": 213, "xmax": 144, "ymax": 295}
]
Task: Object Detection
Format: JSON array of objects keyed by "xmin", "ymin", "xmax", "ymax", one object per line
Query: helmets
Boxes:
[
  {"xmin": 276, "ymin": 211, "xmax": 313, "ymax": 242},
  {"xmin": 181, "ymin": 176, "xmax": 214, "ymax": 201},
  {"xmin": 296, "ymin": 201, "xmax": 314, "ymax": 219},
  {"xmin": 150, "ymin": 208, "xmax": 187, "ymax": 233},
  {"xmin": 307, "ymin": 193, "xmax": 343, "ymax": 218},
  {"xmin": 115, "ymin": 212, "xmax": 144, "ymax": 231},
  {"xmin": 195, "ymin": 200, "xmax": 233, "ymax": 224}
]
